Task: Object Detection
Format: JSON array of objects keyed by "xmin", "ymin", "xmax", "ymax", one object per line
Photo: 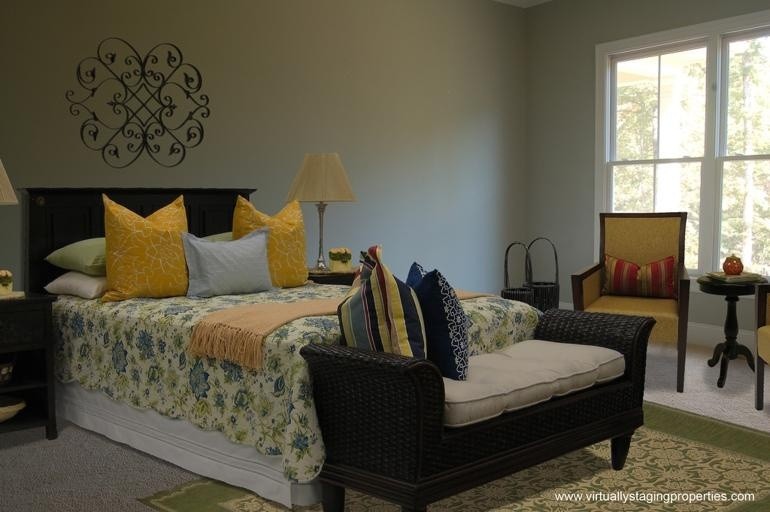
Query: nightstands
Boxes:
[
  {"xmin": 0, "ymin": 291, "xmax": 58, "ymax": 441},
  {"xmin": 308, "ymin": 268, "xmax": 362, "ymax": 286}
]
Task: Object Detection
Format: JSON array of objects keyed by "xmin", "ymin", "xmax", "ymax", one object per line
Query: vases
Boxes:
[
  {"xmin": 0, "ymin": 284, "xmax": 13, "ymax": 297},
  {"xmin": 331, "ymin": 257, "xmax": 352, "ymax": 273}
]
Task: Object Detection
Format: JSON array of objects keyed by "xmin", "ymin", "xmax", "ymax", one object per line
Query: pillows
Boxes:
[
  {"xmin": 180, "ymin": 225, "xmax": 275, "ymax": 297},
  {"xmin": 232, "ymin": 194, "xmax": 309, "ymax": 288},
  {"xmin": 338, "ymin": 244, "xmax": 427, "ymax": 364},
  {"xmin": 600, "ymin": 252, "xmax": 677, "ymax": 300},
  {"xmin": 101, "ymin": 192, "xmax": 190, "ymax": 301},
  {"xmin": 198, "ymin": 230, "xmax": 234, "ymax": 242},
  {"xmin": 406, "ymin": 259, "xmax": 471, "ymax": 382},
  {"xmin": 44, "ymin": 270, "xmax": 108, "ymax": 300},
  {"xmin": 45, "ymin": 236, "xmax": 107, "ymax": 277}
]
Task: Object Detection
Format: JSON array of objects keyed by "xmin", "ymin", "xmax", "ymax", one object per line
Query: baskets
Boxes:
[
  {"xmin": 523, "ymin": 237, "xmax": 559, "ymax": 311},
  {"xmin": 501, "ymin": 243, "xmax": 534, "ymax": 307}
]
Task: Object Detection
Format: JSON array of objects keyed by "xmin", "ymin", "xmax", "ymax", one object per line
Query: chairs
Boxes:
[
  {"xmin": 567, "ymin": 211, "xmax": 691, "ymax": 393},
  {"xmin": 755, "ymin": 283, "xmax": 770, "ymax": 412}
]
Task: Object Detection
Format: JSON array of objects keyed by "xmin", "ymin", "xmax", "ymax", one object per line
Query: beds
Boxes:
[
  {"xmin": 19, "ymin": 186, "xmax": 546, "ymax": 504},
  {"xmin": 696, "ymin": 272, "xmax": 768, "ymax": 389}
]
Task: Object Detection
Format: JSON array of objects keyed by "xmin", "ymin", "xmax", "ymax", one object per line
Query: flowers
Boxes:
[
  {"xmin": 0, "ymin": 269, "xmax": 14, "ymax": 288},
  {"xmin": 328, "ymin": 247, "xmax": 353, "ymax": 264}
]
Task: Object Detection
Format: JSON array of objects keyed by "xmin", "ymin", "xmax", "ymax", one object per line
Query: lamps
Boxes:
[
  {"xmin": 0, "ymin": 156, "xmax": 20, "ymax": 205},
  {"xmin": 285, "ymin": 152, "xmax": 356, "ymax": 272}
]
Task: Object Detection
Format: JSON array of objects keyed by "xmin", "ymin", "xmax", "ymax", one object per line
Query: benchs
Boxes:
[{"xmin": 297, "ymin": 307, "xmax": 658, "ymax": 511}]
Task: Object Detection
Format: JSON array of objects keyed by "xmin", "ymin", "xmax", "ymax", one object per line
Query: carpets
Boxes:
[{"xmin": 135, "ymin": 399, "xmax": 769, "ymax": 511}]
